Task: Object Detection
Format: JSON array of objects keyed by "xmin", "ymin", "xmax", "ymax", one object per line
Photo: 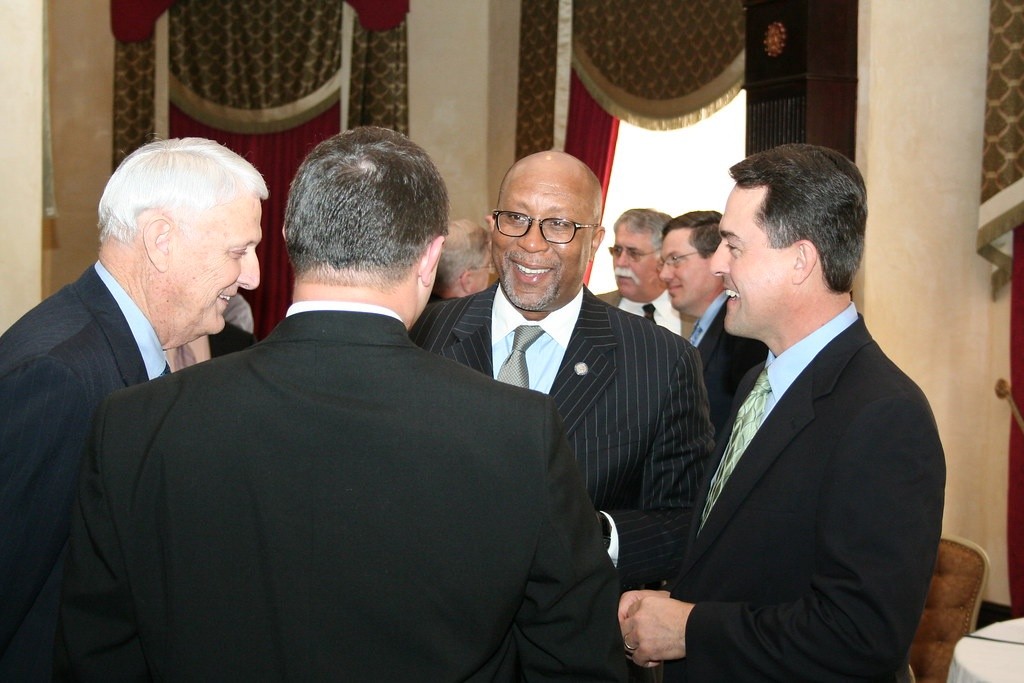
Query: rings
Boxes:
[{"xmin": 624, "ymin": 634, "xmax": 637, "ymax": 651}]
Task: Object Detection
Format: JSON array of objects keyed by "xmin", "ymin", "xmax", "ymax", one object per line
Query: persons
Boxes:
[
  {"xmin": 409, "ymin": 150, "xmax": 769, "ymax": 683},
  {"xmin": 619, "ymin": 142, "xmax": 947, "ymax": 683},
  {"xmin": 55, "ymin": 127, "xmax": 628, "ymax": 682},
  {"xmin": 0, "ymin": 136, "xmax": 269, "ymax": 683}
]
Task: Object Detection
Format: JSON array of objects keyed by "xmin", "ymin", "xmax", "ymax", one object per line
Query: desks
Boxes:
[{"xmin": 946, "ymin": 618, "xmax": 1024, "ymax": 683}]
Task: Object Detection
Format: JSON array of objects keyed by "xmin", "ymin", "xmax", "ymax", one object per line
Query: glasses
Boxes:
[
  {"xmin": 493, "ymin": 210, "xmax": 599, "ymax": 244},
  {"xmin": 609, "ymin": 247, "xmax": 661, "ymax": 262},
  {"xmin": 656, "ymin": 249, "xmax": 715, "ymax": 271},
  {"xmin": 460, "ymin": 261, "xmax": 495, "ymax": 279}
]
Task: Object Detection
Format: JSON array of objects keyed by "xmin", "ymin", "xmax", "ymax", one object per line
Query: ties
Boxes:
[
  {"xmin": 497, "ymin": 325, "xmax": 545, "ymax": 389},
  {"xmin": 160, "ymin": 361, "xmax": 171, "ymax": 377},
  {"xmin": 643, "ymin": 304, "xmax": 657, "ymax": 324},
  {"xmin": 689, "ymin": 321, "xmax": 703, "ymax": 345},
  {"xmin": 697, "ymin": 366, "xmax": 771, "ymax": 537}
]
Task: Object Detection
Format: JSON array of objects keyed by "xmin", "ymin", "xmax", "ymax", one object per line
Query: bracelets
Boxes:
[{"xmin": 599, "ymin": 513, "xmax": 611, "ymax": 548}]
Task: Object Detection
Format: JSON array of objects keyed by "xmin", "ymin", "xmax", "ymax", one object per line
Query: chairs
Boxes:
[{"xmin": 909, "ymin": 534, "xmax": 992, "ymax": 683}]
